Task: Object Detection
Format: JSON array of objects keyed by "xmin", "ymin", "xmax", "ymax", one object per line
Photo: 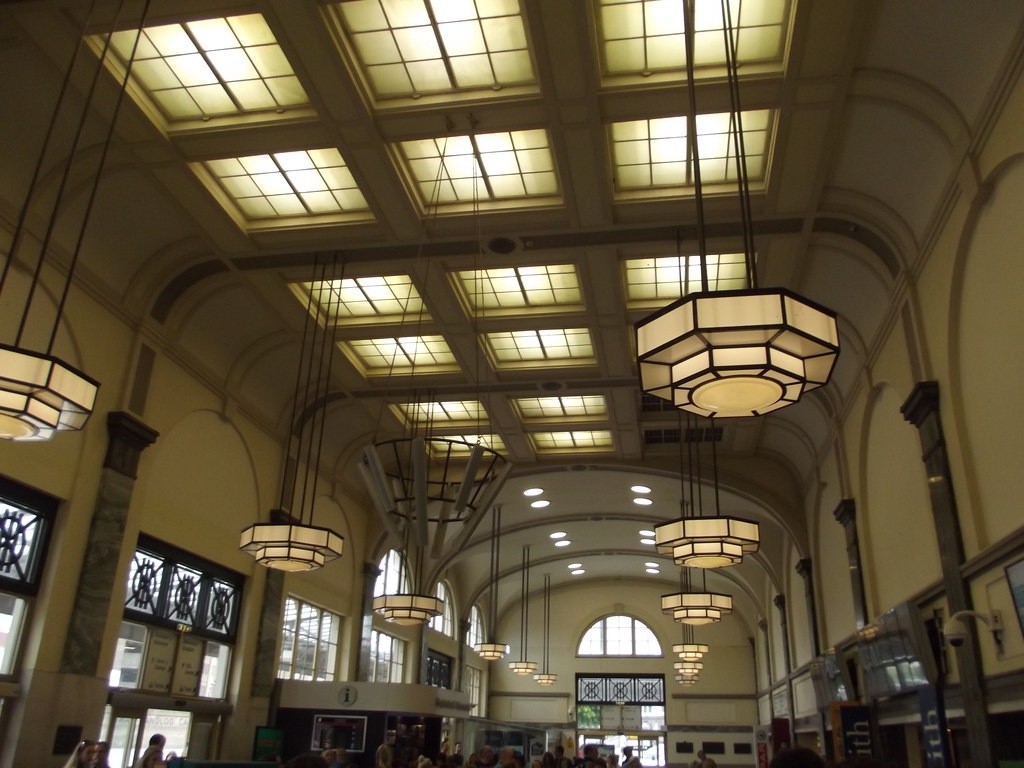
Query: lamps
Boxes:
[
  {"xmin": 372, "ymin": 391, "xmax": 445, "ymax": 627},
  {"xmin": 660, "ymin": 501, "xmax": 734, "ymax": 627},
  {"xmin": 239, "ymin": 249, "xmax": 347, "ymax": 575},
  {"xmin": 653, "ymin": 409, "xmax": 762, "ymax": 571},
  {"xmin": 533, "ymin": 576, "xmax": 559, "ymax": 686},
  {"xmin": 472, "ymin": 506, "xmax": 512, "ymax": 661},
  {"xmin": 508, "ymin": 545, "xmax": 539, "ymax": 677},
  {"xmin": 632, "ymin": 0, "xmax": 842, "ymax": 419},
  {"xmin": 1, "ymin": 1, "xmax": 154, "ymax": 443},
  {"xmin": 672, "ymin": 567, "xmax": 709, "ymax": 688}
]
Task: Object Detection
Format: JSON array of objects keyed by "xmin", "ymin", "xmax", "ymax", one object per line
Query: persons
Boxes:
[
  {"xmin": 135, "ymin": 733, "xmax": 167, "ymax": 768},
  {"xmin": 88, "ymin": 741, "xmax": 112, "ymax": 768},
  {"xmin": 698, "ymin": 750, "xmax": 717, "ymax": 768},
  {"xmin": 63, "ymin": 739, "xmax": 95, "ymax": 768},
  {"xmin": 767, "ymin": 746, "xmax": 826, "ymax": 768},
  {"xmin": 251, "ymin": 740, "xmax": 644, "ymax": 768},
  {"xmin": 164, "ymin": 750, "xmax": 178, "ymax": 762}
]
